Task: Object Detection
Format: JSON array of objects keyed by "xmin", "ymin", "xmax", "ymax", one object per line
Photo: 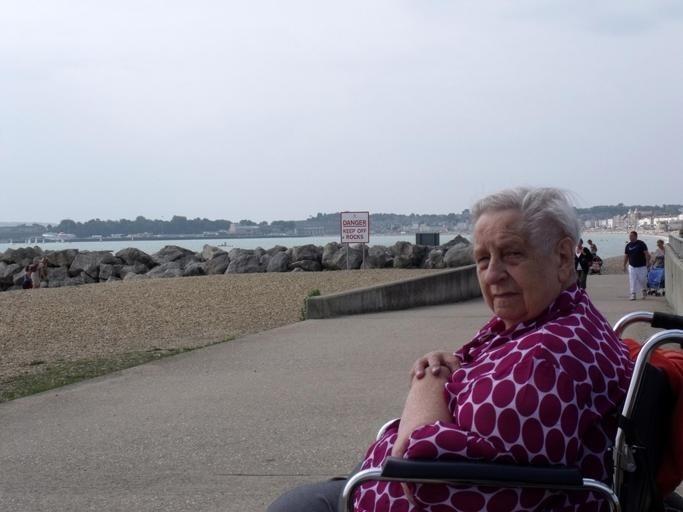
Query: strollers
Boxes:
[
  {"xmin": 645, "ymin": 257, "xmax": 666, "ymax": 296},
  {"xmin": 587, "ymin": 254, "xmax": 603, "ymax": 275}
]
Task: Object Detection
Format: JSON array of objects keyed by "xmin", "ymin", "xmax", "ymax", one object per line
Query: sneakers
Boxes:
[
  {"xmin": 640, "ymin": 291, "xmax": 645, "ymax": 299},
  {"xmin": 630, "ymin": 294, "xmax": 636, "ymax": 299}
]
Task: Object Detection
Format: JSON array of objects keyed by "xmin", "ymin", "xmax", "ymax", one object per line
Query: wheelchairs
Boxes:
[{"xmin": 340, "ymin": 309, "xmax": 682, "ymax": 512}]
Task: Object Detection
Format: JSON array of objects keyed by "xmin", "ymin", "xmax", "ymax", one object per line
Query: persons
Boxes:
[
  {"xmin": 21, "ymin": 271, "xmax": 32, "ymax": 289},
  {"xmin": 572, "ymin": 237, "xmax": 593, "ymax": 291},
  {"xmin": 261, "ymin": 185, "xmax": 636, "ymax": 510},
  {"xmin": 587, "ymin": 240, "xmax": 597, "ymax": 275},
  {"xmin": 621, "ymin": 231, "xmax": 650, "ymax": 301},
  {"xmin": 649, "ymin": 239, "xmax": 665, "ymax": 296}
]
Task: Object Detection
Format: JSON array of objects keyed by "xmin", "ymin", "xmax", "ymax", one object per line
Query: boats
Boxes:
[{"xmin": 217, "ymin": 241, "xmax": 233, "ymax": 247}]
